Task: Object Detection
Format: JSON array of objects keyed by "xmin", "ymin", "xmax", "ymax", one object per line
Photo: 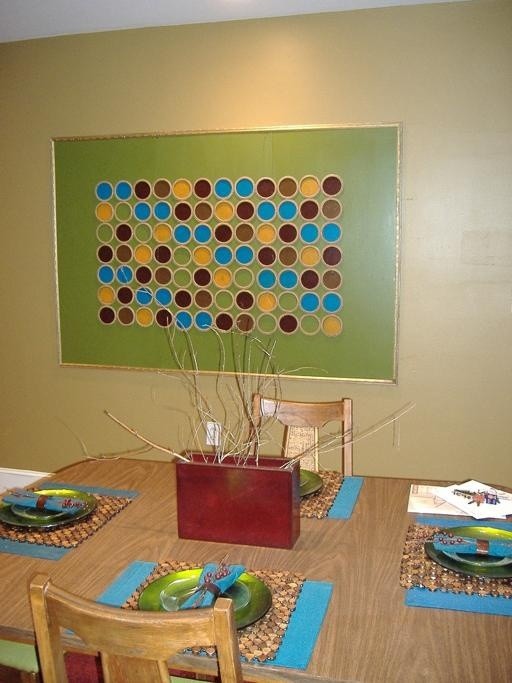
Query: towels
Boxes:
[
  {"xmin": 2, "ymin": 482, "xmax": 90, "ymax": 518},
  {"xmin": 165, "ymin": 560, "xmax": 248, "ymax": 615},
  {"xmin": 426, "ymin": 526, "xmax": 511, "ymax": 558}
]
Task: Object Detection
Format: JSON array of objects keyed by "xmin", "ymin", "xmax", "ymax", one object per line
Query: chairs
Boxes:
[
  {"xmin": 22, "ymin": 571, "xmax": 246, "ymax": 683},
  {"xmin": 244, "ymin": 388, "xmax": 355, "ymax": 480}
]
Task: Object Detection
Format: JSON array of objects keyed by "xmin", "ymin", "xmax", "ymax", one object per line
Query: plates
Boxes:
[
  {"xmin": 423, "ymin": 525, "xmax": 511, "ymax": 579},
  {"xmin": 297, "ymin": 466, "xmax": 323, "ymax": 499},
  {"xmin": 136, "ymin": 568, "xmax": 274, "ymax": 627},
  {"xmin": 1, "ymin": 488, "xmax": 97, "ymax": 527}
]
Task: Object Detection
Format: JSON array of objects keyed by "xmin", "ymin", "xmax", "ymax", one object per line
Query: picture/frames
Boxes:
[{"xmin": 42, "ymin": 119, "xmax": 405, "ymax": 387}]
